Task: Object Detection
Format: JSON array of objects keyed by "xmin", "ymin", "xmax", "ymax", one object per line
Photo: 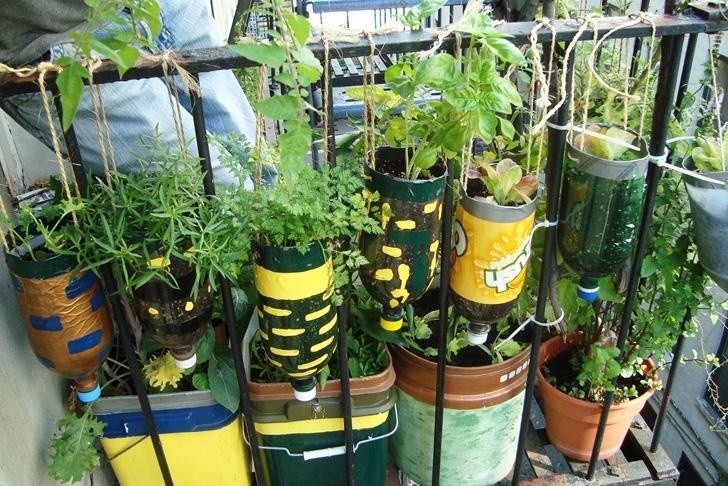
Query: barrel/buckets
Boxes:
[
  {"xmin": 389, "ymin": 340, "xmax": 535, "ymax": 486},
  {"xmin": 242, "ymin": 382, "xmax": 400, "ymax": 486},
  {"xmin": 82, "ymin": 404, "xmax": 256, "ymax": 486}
]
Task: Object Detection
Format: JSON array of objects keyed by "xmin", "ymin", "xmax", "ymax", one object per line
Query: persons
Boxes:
[{"xmin": 0, "ymin": 1, "xmax": 277, "ymax": 197}]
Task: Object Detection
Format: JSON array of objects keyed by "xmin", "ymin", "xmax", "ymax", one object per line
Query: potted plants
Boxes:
[{"xmin": 0, "ymin": 0, "xmax": 727, "ymax": 486}]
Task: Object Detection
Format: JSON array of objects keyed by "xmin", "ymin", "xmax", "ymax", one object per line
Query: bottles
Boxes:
[
  {"xmin": 124, "ymin": 234, "xmax": 215, "ymax": 370},
  {"xmin": 356, "ymin": 144, "xmax": 448, "ymax": 333},
  {"xmin": 557, "ymin": 121, "xmax": 650, "ymax": 303},
  {"xmin": 449, "ymin": 162, "xmax": 539, "ymax": 346},
  {"xmin": 2, "ymin": 213, "xmax": 115, "ymax": 406},
  {"xmin": 251, "ymin": 236, "xmax": 340, "ymax": 403}
]
